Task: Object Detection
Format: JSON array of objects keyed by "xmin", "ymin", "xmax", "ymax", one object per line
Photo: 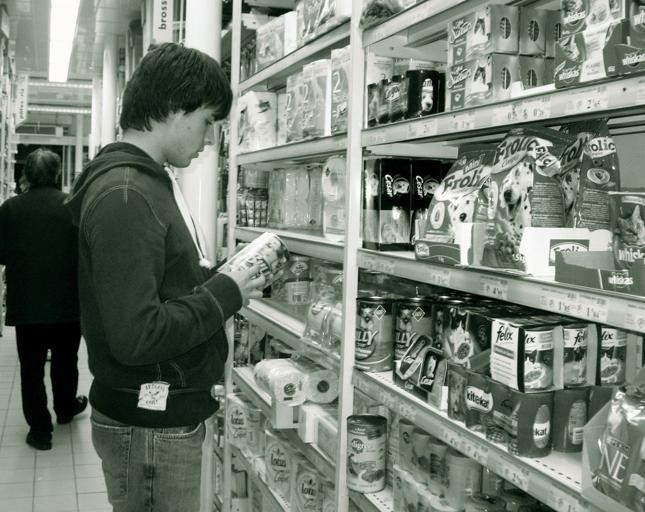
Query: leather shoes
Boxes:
[
  {"xmin": 25, "ymin": 426, "xmax": 53, "ymax": 449},
  {"xmin": 57, "ymin": 394, "xmax": 87, "ymax": 424}
]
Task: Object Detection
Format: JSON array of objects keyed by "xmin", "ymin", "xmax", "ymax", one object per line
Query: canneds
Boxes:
[
  {"xmin": 224, "ymin": 252, "xmax": 625, "ymax": 512},
  {"xmin": 368, "ymin": 70, "xmax": 445, "ymax": 126}
]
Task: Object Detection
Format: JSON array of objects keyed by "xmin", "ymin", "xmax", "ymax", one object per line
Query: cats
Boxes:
[
  {"xmin": 614, "ymin": 204, "xmax": 645, "ymax": 246},
  {"xmin": 447, "ymin": 307, "xmax": 473, "ymax": 369}
]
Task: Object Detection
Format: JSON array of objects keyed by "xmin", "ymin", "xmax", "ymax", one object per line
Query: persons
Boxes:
[
  {"xmin": 0, "ymin": 149, "xmax": 87, "ymax": 450},
  {"xmin": 63, "ymin": 43, "xmax": 266, "ymax": 512}
]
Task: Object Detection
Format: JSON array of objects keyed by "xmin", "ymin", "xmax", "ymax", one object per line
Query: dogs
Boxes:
[
  {"xmin": 448, "ymin": 193, "xmax": 477, "ymax": 266},
  {"xmin": 499, "ymin": 161, "xmax": 534, "ymax": 229}
]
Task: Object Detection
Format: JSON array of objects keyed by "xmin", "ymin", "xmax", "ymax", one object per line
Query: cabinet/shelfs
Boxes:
[
  {"xmin": 0, "ymin": 9, "xmax": 18, "ymax": 335},
  {"xmin": 112, "ymin": 1, "xmax": 644, "ymax": 512}
]
